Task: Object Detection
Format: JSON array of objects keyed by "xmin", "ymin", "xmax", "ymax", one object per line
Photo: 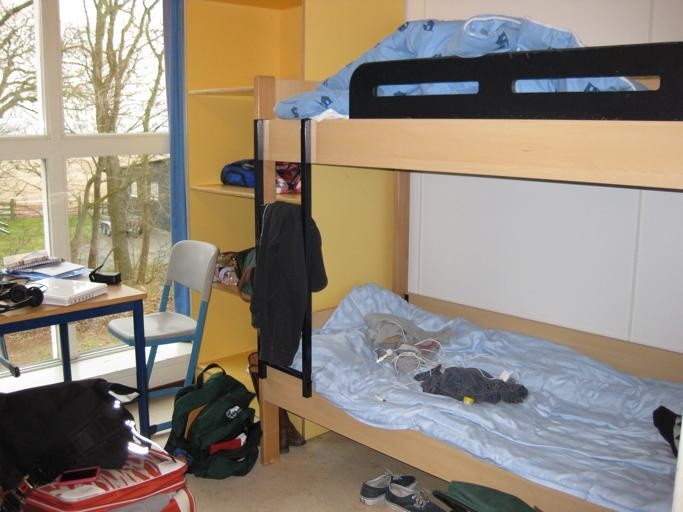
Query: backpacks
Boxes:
[{"xmin": 165, "ymin": 363, "xmax": 258, "ymax": 479}]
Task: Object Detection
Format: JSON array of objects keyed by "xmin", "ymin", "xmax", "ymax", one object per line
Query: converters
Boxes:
[{"xmin": 500, "ymin": 368, "xmax": 514, "ymax": 382}]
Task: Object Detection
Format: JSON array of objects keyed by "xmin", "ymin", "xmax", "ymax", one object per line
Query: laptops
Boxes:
[{"xmin": 26, "ymin": 276, "xmax": 108, "ymax": 307}]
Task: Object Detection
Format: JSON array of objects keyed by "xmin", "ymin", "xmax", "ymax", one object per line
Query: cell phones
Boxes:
[{"xmin": 56, "ymin": 466, "xmax": 101, "ymax": 486}]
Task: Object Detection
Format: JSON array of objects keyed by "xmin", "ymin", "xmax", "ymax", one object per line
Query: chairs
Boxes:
[{"xmin": 107, "ymin": 239, "xmax": 219, "ymax": 435}]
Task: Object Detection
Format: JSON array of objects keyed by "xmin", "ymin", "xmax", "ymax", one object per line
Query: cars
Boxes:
[{"xmin": 100, "ymin": 211, "xmax": 145, "ymax": 236}]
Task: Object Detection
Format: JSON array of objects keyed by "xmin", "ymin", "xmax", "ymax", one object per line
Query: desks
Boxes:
[{"xmin": 0, "ymin": 266, "xmax": 149, "ymax": 444}]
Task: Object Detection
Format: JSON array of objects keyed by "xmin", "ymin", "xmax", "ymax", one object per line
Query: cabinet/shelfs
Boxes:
[{"xmin": 181, "ymin": 0, "xmax": 403, "ymax": 445}]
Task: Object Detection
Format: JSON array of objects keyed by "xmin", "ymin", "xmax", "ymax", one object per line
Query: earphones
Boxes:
[{"xmin": 376, "ymin": 349, "xmax": 393, "ymax": 363}]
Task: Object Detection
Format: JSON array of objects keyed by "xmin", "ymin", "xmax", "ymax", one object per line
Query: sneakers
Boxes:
[
  {"xmin": 384, "ymin": 483, "xmax": 447, "ymax": 512},
  {"xmin": 360, "ymin": 467, "xmax": 418, "ymax": 506}
]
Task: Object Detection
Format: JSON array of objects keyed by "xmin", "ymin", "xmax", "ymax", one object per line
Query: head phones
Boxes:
[{"xmin": 0, "ymin": 284, "xmax": 44, "ymax": 311}]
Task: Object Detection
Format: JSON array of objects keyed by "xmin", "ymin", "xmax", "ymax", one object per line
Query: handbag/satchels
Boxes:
[
  {"xmin": 221, "ymin": 160, "xmax": 255, "ymax": 187},
  {"xmin": 16, "ymin": 378, "xmax": 148, "ymax": 484}
]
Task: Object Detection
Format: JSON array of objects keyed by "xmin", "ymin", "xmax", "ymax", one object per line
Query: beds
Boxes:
[{"xmin": 254, "ymin": 40, "xmax": 683, "ymax": 512}]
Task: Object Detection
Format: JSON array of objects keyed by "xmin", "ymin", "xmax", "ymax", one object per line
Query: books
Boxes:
[{"xmin": 4, "ymin": 258, "xmax": 90, "ymax": 280}]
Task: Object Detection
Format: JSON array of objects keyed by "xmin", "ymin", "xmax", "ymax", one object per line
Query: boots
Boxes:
[{"xmin": 248, "ymin": 352, "xmax": 306, "ymax": 454}]
